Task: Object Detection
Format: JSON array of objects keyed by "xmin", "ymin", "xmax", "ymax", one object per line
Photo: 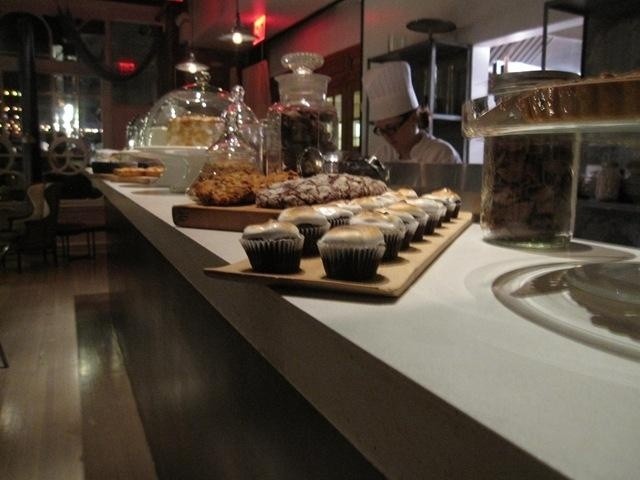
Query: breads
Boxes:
[
  {"xmin": 113, "ymin": 166, "xmax": 163, "ymax": 177},
  {"xmin": 190, "ymin": 160, "xmax": 388, "ymax": 208}
]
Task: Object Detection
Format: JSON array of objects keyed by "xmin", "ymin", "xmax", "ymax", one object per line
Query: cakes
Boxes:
[
  {"xmin": 242, "ymin": 186, "xmax": 461, "ymax": 280},
  {"xmin": 168, "ymin": 116, "xmax": 226, "ymax": 147}
]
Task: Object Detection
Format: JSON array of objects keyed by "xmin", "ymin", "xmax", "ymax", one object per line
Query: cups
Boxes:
[{"xmin": 240, "ymin": 190, "xmax": 461, "ymax": 277}]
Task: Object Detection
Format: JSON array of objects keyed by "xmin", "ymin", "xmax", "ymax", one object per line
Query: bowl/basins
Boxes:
[
  {"xmin": 567, "ymin": 261, "xmax": 640, "ymax": 329},
  {"xmin": 135, "ymin": 145, "xmax": 210, "ymax": 190}
]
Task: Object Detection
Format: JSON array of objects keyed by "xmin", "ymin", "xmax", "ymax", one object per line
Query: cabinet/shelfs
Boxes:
[
  {"xmin": 367, "ymin": 39, "xmax": 472, "ymax": 190},
  {"xmin": 541, "ymin": 0, "xmax": 640, "ymax": 216},
  {"xmin": 459, "ymin": 70, "xmax": 640, "ymax": 241}
]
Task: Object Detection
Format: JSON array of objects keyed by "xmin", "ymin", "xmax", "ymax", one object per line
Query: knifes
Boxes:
[{"xmin": 511, "ymin": 264, "xmax": 569, "ymax": 296}]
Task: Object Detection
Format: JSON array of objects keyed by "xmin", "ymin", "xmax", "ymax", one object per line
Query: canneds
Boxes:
[{"xmin": 480, "ymin": 71, "xmax": 583, "ymax": 250}]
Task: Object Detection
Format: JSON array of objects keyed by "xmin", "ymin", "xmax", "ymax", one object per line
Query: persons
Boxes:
[
  {"xmin": 362, "ymin": 61, "xmax": 465, "ymax": 194},
  {"xmin": 20, "ymin": 132, "xmax": 103, "ymax": 256}
]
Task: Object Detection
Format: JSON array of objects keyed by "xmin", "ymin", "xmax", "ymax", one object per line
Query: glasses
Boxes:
[{"xmin": 374, "ymin": 111, "xmax": 408, "ymax": 136}]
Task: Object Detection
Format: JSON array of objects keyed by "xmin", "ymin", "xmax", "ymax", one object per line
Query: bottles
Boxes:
[
  {"xmin": 187, "ymin": 109, "xmax": 264, "ymax": 205},
  {"xmin": 595, "ymin": 160, "xmax": 621, "ymax": 202},
  {"xmin": 255, "ymin": 117, "xmax": 285, "ymax": 180},
  {"xmin": 265, "ymin": 50, "xmax": 339, "ymax": 178},
  {"xmin": 214, "ymin": 83, "xmax": 264, "ymax": 179}
]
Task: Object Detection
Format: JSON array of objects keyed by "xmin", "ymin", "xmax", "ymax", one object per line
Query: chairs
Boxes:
[{"xmin": 0, "ymin": 171, "xmax": 107, "ymax": 273}]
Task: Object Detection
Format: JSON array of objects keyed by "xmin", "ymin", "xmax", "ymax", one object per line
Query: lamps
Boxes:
[
  {"xmin": 175, "ymin": 0, "xmax": 211, "ymax": 74},
  {"xmin": 217, "ymin": 0, "xmax": 257, "ymax": 44}
]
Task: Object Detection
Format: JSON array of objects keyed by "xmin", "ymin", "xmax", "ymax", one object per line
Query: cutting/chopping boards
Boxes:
[
  {"xmin": 171, "ymin": 201, "xmax": 287, "ymax": 232},
  {"xmin": 95, "ymin": 173, "xmax": 158, "ymax": 184}
]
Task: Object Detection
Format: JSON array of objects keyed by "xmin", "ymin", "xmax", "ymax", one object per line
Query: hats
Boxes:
[{"xmin": 362, "ymin": 61, "xmax": 419, "ymax": 122}]
